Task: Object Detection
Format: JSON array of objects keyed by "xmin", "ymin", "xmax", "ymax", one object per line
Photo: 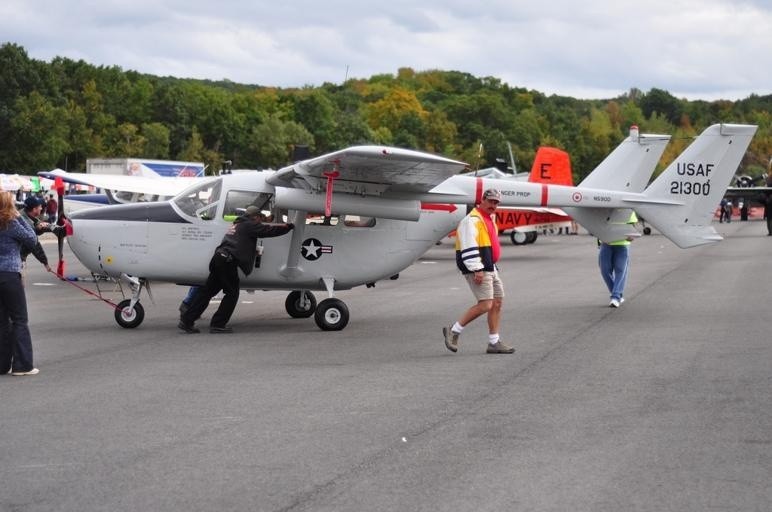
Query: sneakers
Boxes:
[
  {"xmin": 177, "ymin": 321, "xmax": 201, "ymax": 333},
  {"xmin": 486, "ymin": 339, "xmax": 515, "ymax": 353},
  {"xmin": 6, "ymin": 363, "xmax": 40, "ymax": 376},
  {"xmin": 609, "ymin": 296, "xmax": 625, "ymax": 308},
  {"xmin": 208, "ymin": 327, "xmax": 233, "ymax": 333},
  {"xmin": 442, "ymin": 324, "xmax": 460, "ymax": 352}
]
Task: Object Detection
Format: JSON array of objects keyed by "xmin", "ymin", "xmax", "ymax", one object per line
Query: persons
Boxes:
[
  {"xmin": 0, "ymin": 190, "xmax": 40, "ymax": 376},
  {"xmin": 18, "ymin": 190, "xmax": 67, "ymax": 272},
  {"xmin": 598, "ymin": 211, "xmax": 637, "ymax": 308},
  {"xmin": 720, "ymin": 199, "xmax": 733, "ymax": 223},
  {"xmin": 443, "ymin": 188, "xmax": 515, "ymax": 354},
  {"xmin": 763, "ymin": 194, "xmax": 772, "ymax": 235},
  {"xmin": 543, "ymin": 220, "xmax": 579, "ymax": 235},
  {"xmin": 179, "ymin": 204, "xmax": 295, "ymax": 333},
  {"xmin": 179, "ymin": 286, "xmax": 200, "ymax": 313}
]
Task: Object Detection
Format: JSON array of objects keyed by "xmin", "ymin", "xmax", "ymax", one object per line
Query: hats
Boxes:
[
  {"xmin": 483, "ymin": 188, "xmax": 502, "ymax": 203},
  {"xmin": 245, "ymin": 205, "xmax": 266, "ymax": 217},
  {"xmin": 24, "ymin": 196, "xmax": 45, "ymax": 208}
]
